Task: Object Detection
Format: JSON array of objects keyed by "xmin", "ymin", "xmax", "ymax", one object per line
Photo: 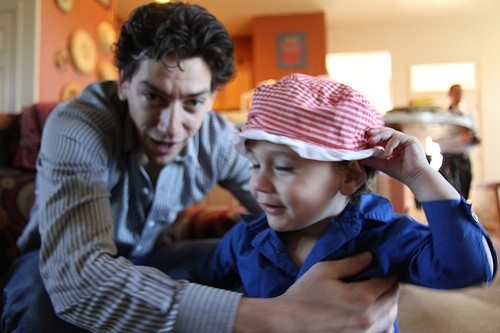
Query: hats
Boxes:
[{"xmin": 238, "ymin": 74, "xmax": 398, "ymax": 164}]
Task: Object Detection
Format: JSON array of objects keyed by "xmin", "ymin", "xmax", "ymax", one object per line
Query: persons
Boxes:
[
  {"xmin": 1, "ymin": 0, "xmax": 401, "ymax": 332},
  {"xmin": 438, "ymin": 84, "xmax": 478, "ymax": 201},
  {"xmin": 204, "ymin": 74, "xmax": 498, "ymax": 332}
]
